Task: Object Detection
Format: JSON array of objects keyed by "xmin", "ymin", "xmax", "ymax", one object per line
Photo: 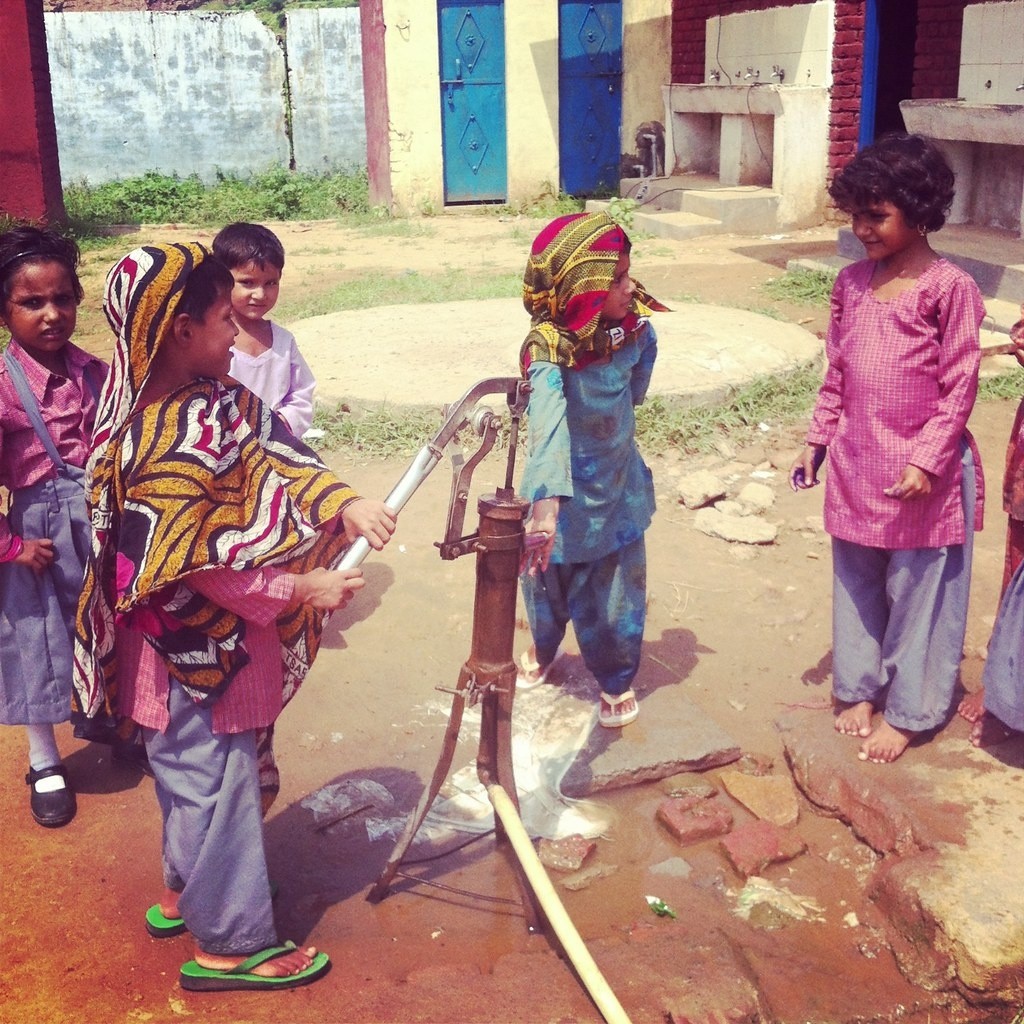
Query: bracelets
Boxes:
[{"xmin": 0, "ymin": 533, "xmax": 24, "ymax": 563}]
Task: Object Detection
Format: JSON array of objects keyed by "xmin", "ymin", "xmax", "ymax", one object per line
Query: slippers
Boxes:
[
  {"xmin": 145, "ymin": 879, "xmax": 278, "ymax": 937},
  {"xmin": 180, "ymin": 936, "xmax": 330, "ymax": 992}
]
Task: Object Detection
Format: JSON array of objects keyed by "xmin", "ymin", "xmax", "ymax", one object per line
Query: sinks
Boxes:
[
  {"xmin": 669, "ymin": 82, "xmax": 818, "ymax": 114},
  {"xmin": 899, "ymin": 98, "xmax": 1024, "ymax": 145}
]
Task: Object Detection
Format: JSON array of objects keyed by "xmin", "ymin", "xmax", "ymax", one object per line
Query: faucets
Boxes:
[
  {"xmin": 769, "ymin": 64, "xmax": 784, "ymax": 78},
  {"xmin": 1017, "ymin": 84, "xmax": 1024, "ymax": 92},
  {"xmin": 709, "ymin": 68, "xmax": 719, "ymax": 80},
  {"xmin": 745, "ymin": 66, "xmax": 760, "ymax": 80}
]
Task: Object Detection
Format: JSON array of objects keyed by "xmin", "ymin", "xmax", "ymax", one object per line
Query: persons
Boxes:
[
  {"xmin": 510, "ymin": 214, "xmax": 675, "ymax": 730},
  {"xmin": 789, "ymin": 129, "xmax": 987, "ymax": 763},
  {"xmin": 0, "ymin": 222, "xmax": 396, "ymax": 993},
  {"xmin": 953, "ymin": 304, "xmax": 1024, "ymax": 748}
]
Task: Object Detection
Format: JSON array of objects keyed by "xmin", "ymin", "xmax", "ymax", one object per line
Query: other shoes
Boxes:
[
  {"xmin": 110, "ymin": 732, "xmax": 156, "ymax": 778},
  {"xmin": 25, "ymin": 765, "xmax": 77, "ymax": 824},
  {"xmin": 73, "ymin": 711, "xmax": 121, "ymax": 745}
]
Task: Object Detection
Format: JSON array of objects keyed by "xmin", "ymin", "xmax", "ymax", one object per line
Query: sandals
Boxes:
[
  {"xmin": 514, "ymin": 641, "xmax": 564, "ymax": 688},
  {"xmin": 597, "ymin": 685, "xmax": 639, "ymax": 727}
]
